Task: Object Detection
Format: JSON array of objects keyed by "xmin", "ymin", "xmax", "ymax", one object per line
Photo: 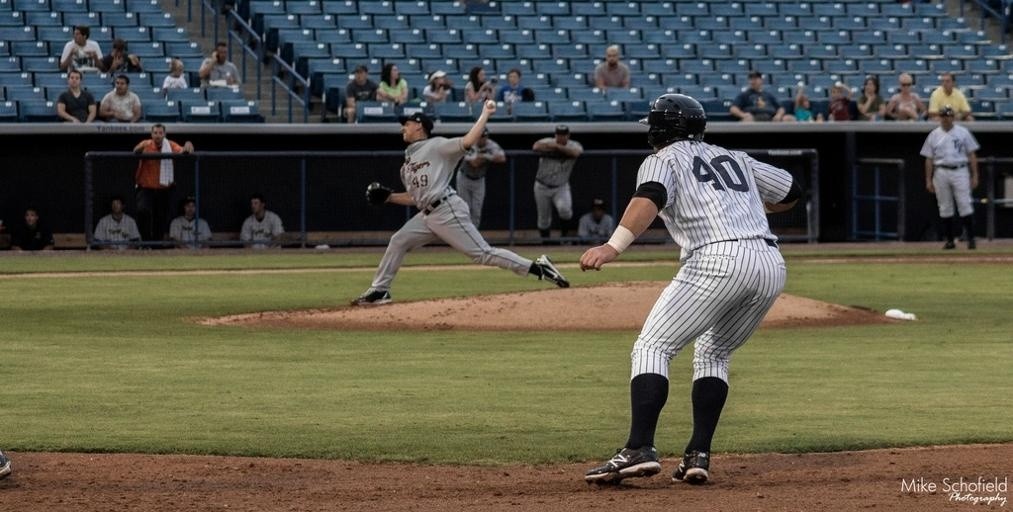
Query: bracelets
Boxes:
[{"xmin": 606, "ymin": 226, "xmax": 636, "ymax": 255}]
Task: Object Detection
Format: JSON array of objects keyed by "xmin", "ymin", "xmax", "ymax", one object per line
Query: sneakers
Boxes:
[
  {"xmin": 944, "ymin": 241, "xmax": 955, "ymax": 249},
  {"xmin": 351, "ymin": 288, "xmax": 392, "ymax": 307},
  {"xmin": 672, "ymin": 450, "xmax": 710, "ymax": 483},
  {"xmin": 967, "ymin": 240, "xmax": 975, "ymax": 250},
  {"xmin": 536, "ymin": 254, "xmax": 569, "ymax": 288},
  {"xmin": 585, "ymin": 444, "xmax": 661, "ymax": 484}
]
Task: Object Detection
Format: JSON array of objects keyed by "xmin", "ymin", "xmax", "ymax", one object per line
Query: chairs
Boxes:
[{"xmin": 2, "ymin": 0, "xmax": 1013, "ymax": 137}]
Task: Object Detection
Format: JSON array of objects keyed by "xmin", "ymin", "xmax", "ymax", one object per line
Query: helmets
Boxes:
[
  {"xmin": 940, "ymin": 105, "xmax": 954, "ymax": 117},
  {"xmin": 639, "ymin": 94, "xmax": 708, "ymax": 149}
]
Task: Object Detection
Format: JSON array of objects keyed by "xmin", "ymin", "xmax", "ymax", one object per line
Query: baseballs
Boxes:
[{"xmin": 487, "ymin": 100, "xmax": 495, "ymax": 108}]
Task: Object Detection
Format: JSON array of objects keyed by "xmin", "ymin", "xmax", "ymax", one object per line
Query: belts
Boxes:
[
  {"xmin": 726, "ymin": 238, "xmax": 778, "ymax": 248},
  {"xmin": 424, "ymin": 193, "xmax": 452, "ymax": 216},
  {"xmin": 535, "ymin": 178, "xmax": 559, "ymax": 189},
  {"xmin": 459, "ymin": 169, "xmax": 484, "ymax": 180},
  {"xmin": 935, "ymin": 163, "xmax": 965, "ymax": 170}
]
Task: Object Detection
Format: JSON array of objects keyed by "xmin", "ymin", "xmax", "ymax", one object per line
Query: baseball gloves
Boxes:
[{"xmin": 366, "ymin": 182, "xmax": 394, "ymax": 207}]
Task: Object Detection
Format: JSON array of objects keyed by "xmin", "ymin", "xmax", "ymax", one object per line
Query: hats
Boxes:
[
  {"xmin": 555, "ymin": 126, "xmax": 569, "ymax": 135},
  {"xmin": 397, "ymin": 113, "xmax": 434, "ymax": 132}
]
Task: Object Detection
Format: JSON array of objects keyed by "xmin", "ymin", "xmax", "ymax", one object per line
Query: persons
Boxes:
[
  {"xmin": 350, "ymin": 100, "xmax": 569, "ymax": 306},
  {"xmin": 57, "ymin": 22, "xmax": 242, "ymax": 124},
  {"xmin": 169, "ymin": 194, "xmax": 213, "ymax": 248},
  {"xmin": 9, "ymin": 208, "xmax": 55, "ymax": 250},
  {"xmin": 921, "ymin": 105, "xmax": 980, "ymax": 250},
  {"xmin": 576, "ymin": 199, "xmax": 613, "ymax": 245},
  {"xmin": 531, "ymin": 125, "xmax": 583, "ymax": 239},
  {"xmin": 457, "ymin": 126, "xmax": 507, "ymax": 229},
  {"xmin": 580, "ymin": 94, "xmax": 802, "ymax": 486},
  {"xmin": 94, "ymin": 196, "xmax": 141, "ymax": 249},
  {"xmin": 239, "ymin": 195, "xmax": 285, "ymax": 249},
  {"xmin": 133, "ymin": 125, "xmax": 194, "ymax": 249}
]
